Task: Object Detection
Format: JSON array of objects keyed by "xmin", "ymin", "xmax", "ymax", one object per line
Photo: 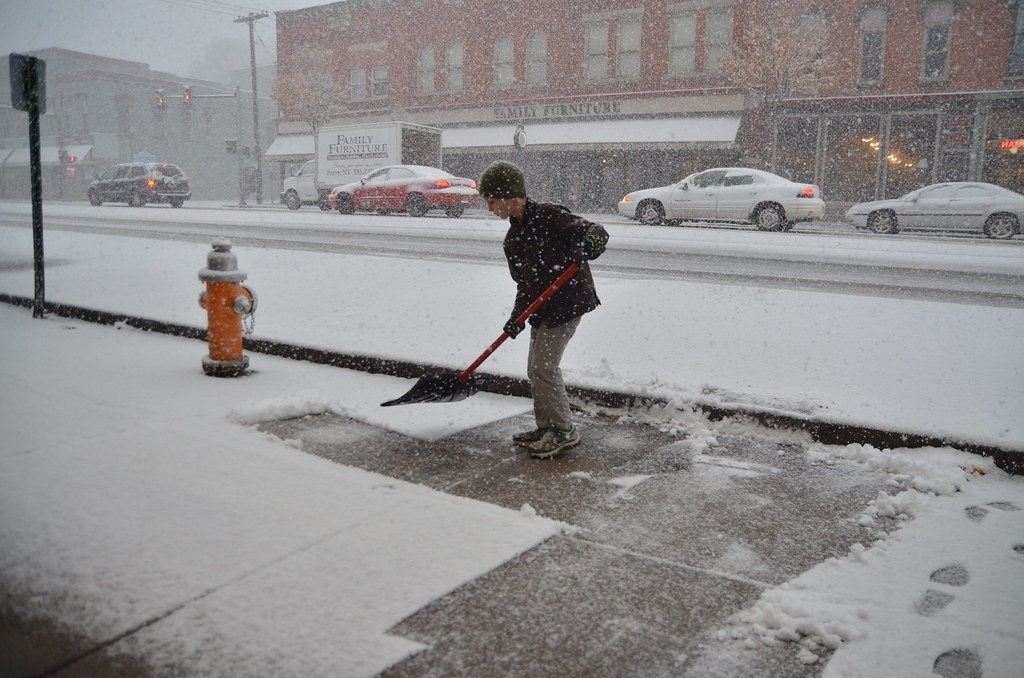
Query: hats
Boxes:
[{"xmin": 479, "ymin": 159, "xmax": 525, "ymax": 199}]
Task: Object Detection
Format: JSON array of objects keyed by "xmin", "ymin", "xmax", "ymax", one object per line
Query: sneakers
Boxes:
[
  {"xmin": 529, "ymin": 424, "xmax": 580, "ymax": 460},
  {"xmin": 513, "ymin": 426, "xmax": 549, "ymax": 447}
]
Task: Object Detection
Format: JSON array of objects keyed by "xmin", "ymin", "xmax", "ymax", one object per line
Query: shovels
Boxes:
[{"xmin": 379, "ymin": 255, "xmax": 596, "ymax": 409}]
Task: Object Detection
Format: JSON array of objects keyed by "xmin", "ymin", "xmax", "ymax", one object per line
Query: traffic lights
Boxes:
[
  {"xmin": 155, "ymin": 89, "xmax": 164, "ymax": 109},
  {"xmin": 184, "ymin": 86, "xmax": 191, "ymax": 106}
]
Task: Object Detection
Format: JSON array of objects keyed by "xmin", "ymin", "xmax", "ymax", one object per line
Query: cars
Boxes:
[
  {"xmin": 327, "ymin": 165, "xmax": 480, "ymax": 220},
  {"xmin": 618, "ymin": 167, "xmax": 825, "ymax": 232},
  {"xmin": 845, "ymin": 181, "xmax": 1024, "ymax": 240}
]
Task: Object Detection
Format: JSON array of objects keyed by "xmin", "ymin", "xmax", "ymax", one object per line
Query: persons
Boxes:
[{"xmin": 479, "ymin": 162, "xmax": 610, "ymax": 457}]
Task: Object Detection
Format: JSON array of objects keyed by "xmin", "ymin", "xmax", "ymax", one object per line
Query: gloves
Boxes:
[
  {"xmin": 574, "ymin": 236, "xmax": 597, "ymax": 265},
  {"xmin": 503, "ymin": 314, "xmax": 525, "ymax": 340}
]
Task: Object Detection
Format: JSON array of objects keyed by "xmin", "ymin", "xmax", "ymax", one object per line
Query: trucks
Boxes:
[{"xmin": 279, "ymin": 119, "xmax": 442, "ymax": 211}]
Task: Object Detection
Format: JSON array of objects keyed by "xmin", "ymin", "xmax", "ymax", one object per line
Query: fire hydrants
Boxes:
[{"xmin": 196, "ymin": 236, "xmax": 259, "ymax": 379}]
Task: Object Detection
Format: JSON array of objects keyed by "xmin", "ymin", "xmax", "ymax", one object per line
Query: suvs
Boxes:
[{"xmin": 87, "ymin": 162, "xmax": 192, "ymax": 208}]
